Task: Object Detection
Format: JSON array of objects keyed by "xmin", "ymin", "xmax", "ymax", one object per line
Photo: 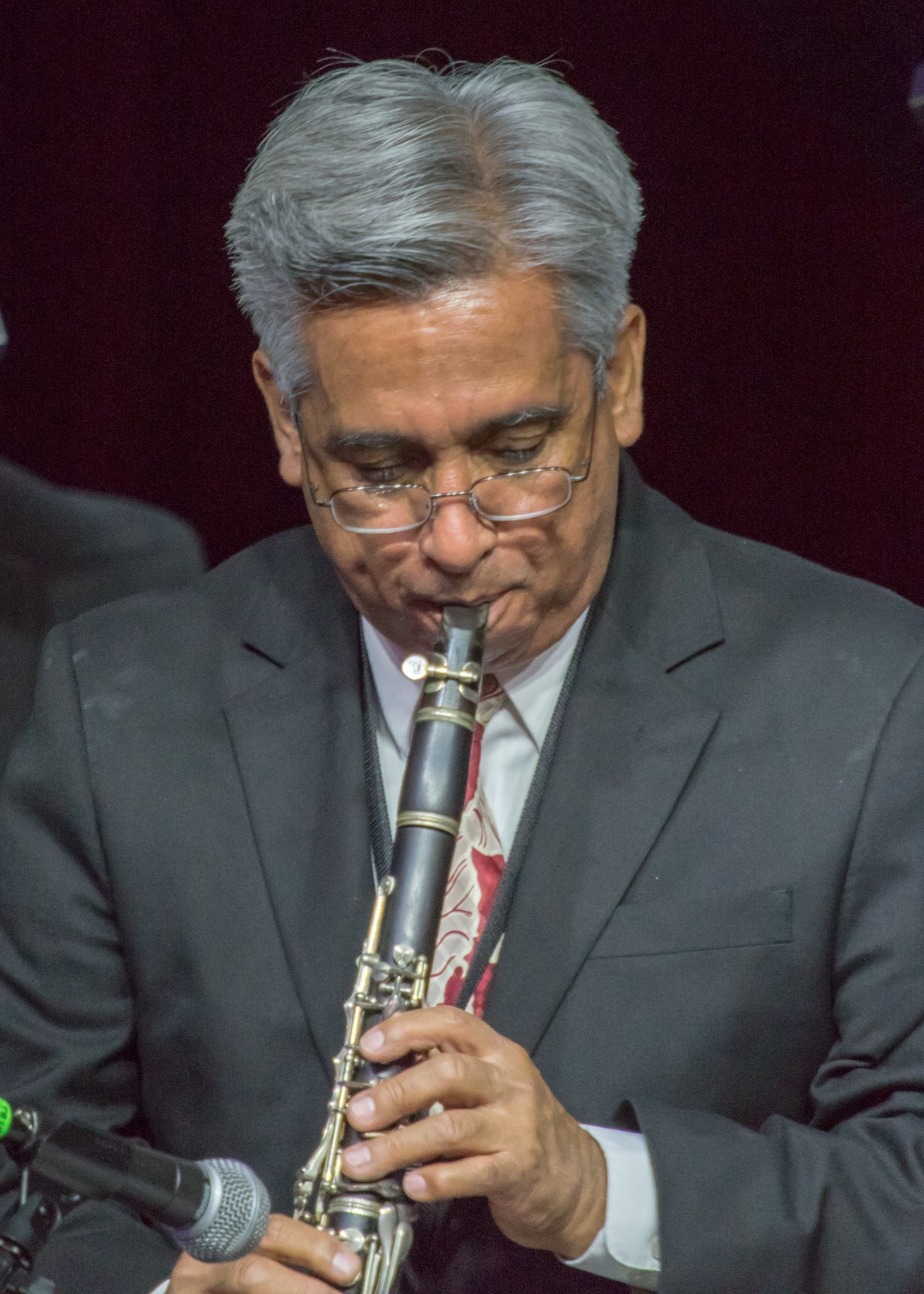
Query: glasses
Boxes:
[{"xmin": 289, "ymin": 353, "xmax": 599, "ymax": 534}]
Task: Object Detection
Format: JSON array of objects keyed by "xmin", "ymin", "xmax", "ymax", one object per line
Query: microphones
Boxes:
[{"xmin": 0, "ymin": 1099, "xmax": 271, "ymax": 1263}]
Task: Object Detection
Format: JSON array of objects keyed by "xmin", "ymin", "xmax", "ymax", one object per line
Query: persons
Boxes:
[{"xmin": 0, "ymin": 59, "xmax": 924, "ymax": 1293}]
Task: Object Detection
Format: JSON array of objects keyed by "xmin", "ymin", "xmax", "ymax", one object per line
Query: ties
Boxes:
[{"xmin": 409, "ymin": 671, "xmax": 508, "ymax": 1278}]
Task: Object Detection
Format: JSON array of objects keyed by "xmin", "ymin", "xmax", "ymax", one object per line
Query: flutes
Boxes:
[{"xmin": 291, "ymin": 599, "xmax": 492, "ymax": 1294}]
